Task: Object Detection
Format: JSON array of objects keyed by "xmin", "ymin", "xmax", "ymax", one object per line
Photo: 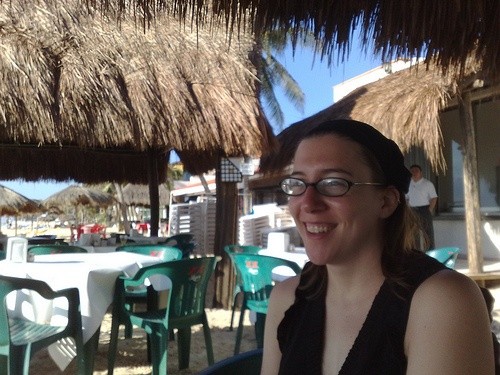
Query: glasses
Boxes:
[{"xmin": 277, "ymin": 176, "xmax": 386, "ymax": 198}]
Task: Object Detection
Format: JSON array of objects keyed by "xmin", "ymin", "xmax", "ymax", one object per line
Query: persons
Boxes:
[
  {"xmin": 405, "ymin": 165, "xmax": 438, "ymax": 252},
  {"xmin": 260, "ymin": 118, "xmax": 494, "ymax": 375}
]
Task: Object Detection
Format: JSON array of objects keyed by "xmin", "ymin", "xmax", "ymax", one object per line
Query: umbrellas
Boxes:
[{"xmin": 0, "ymin": 184, "xmax": 170, "ymax": 241}]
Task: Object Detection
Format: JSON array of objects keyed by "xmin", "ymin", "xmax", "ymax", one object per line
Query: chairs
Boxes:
[
  {"xmin": 0, "ymin": 275, "xmax": 85, "ymax": 375},
  {"xmin": 115, "ymin": 244, "xmax": 184, "ymax": 339},
  {"xmin": 229, "ymin": 253, "xmax": 302, "ymax": 355},
  {"xmin": 108, "ymin": 256, "xmax": 218, "ymax": 375},
  {"xmin": 224, "ymin": 242, "xmax": 266, "ymax": 330},
  {"xmin": 423, "ymin": 246, "xmax": 460, "ymax": 271}
]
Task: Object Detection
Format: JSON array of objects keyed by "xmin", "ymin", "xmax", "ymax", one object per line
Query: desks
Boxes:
[
  {"xmin": 257, "ymin": 247, "xmax": 311, "ymax": 283},
  {"xmin": 3, "ymin": 252, "xmax": 172, "ymax": 371}
]
